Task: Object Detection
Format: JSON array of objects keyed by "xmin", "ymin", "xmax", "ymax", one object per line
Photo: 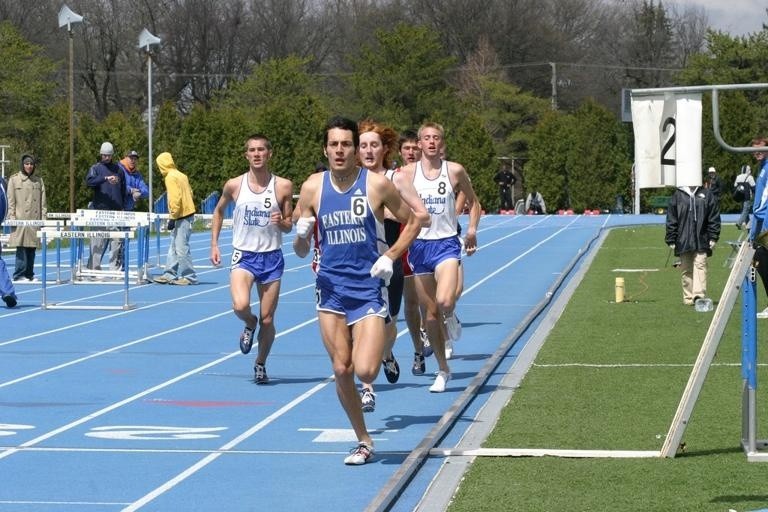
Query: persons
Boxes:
[
  {"xmin": 665, "ymin": 186, "xmax": 721, "ymax": 306},
  {"xmin": 117, "ymin": 150, "xmax": 148, "ymax": 231},
  {"xmin": 208, "ymin": 132, "xmax": 293, "ymax": 384},
  {"xmin": 6, "ymin": 155, "xmax": 48, "ymax": 282},
  {"xmin": 733, "ymin": 164, "xmax": 756, "ymax": 230},
  {"xmin": 525, "ymin": 191, "xmax": 548, "ymax": 215},
  {"xmin": 0, "ymin": 174, "xmax": 19, "ymax": 308},
  {"xmin": 84, "ymin": 142, "xmax": 126, "ymax": 282},
  {"xmin": 748, "ymin": 138, "xmax": 768, "ymax": 318},
  {"xmin": 492, "ymin": 165, "xmax": 517, "ymax": 211},
  {"xmin": 704, "ymin": 166, "xmax": 721, "ymax": 208},
  {"xmin": 152, "ymin": 152, "xmax": 198, "ymax": 285}
]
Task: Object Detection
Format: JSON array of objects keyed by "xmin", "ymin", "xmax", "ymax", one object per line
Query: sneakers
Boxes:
[
  {"xmin": 112, "ymin": 271, "xmax": 124, "ymax": 281},
  {"xmin": 2, "ymin": 293, "xmax": 18, "ymax": 307},
  {"xmin": 87, "ymin": 276, "xmax": 107, "ymax": 282},
  {"xmin": 683, "ymin": 295, "xmax": 705, "ymax": 306},
  {"xmin": 411, "ymin": 351, "xmax": 426, "ymax": 376},
  {"xmin": 239, "ymin": 314, "xmax": 258, "ymax": 355},
  {"xmin": 420, "ymin": 327, "xmax": 434, "ymax": 357},
  {"xmin": 153, "ymin": 275, "xmax": 172, "ymax": 284},
  {"xmin": 756, "ymin": 307, "xmax": 768, "ymax": 318},
  {"xmin": 444, "ymin": 337, "xmax": 453, "ymax": 359},
  {"xmin": 360, "ymin": 387, "xmax": 376, "ymax": 412},
  {"xmin": 168, "ymin": 277, "xmax": 192, "ymax": 285},
  {"xmin": 429, "ymin": 365, "xmax": 452, "ymax": 393},
  {"xmin": 14, "ymin": 274, "xmax": 38, "ymax": 282},
  {"xmin": 344, "ymin": 441, "xmax": 378, "ymax": 465},
  {"xmin": 254, "ymin": 359, "xmax": 270, "ymax": 385},
  {"xmin": 382, "ymin": 351, "xmax": 400, "ymax": 383},
  {"xmin": 443, "ymin": 310, "xmax": 462, "ymax": 340}
]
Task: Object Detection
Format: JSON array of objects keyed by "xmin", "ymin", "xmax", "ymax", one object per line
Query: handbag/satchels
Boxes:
[{"xmin": 732, "ymin": 185, "xmax": 745, "ymax": 203}]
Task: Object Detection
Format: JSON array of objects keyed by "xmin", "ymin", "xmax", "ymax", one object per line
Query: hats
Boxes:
[
  {"xmin": 99, "ymin": 142, "xmax": 114, "ymax": 155},
  {"xmin": 708, "ymin": 167, "xmax": 715, "ymax": 173},
  {"xmin": 23, "ymin": 157, "xmax": 33, "ymax": 163},
  {"xmin": 127, "ymin": 150, "xmax": 139, "ymax": 159}
]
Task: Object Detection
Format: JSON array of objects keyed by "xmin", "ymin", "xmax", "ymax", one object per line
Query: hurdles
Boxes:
[{"xmin": 2, "ymin": 208, "xmax": 162, "ymax": 309}]
